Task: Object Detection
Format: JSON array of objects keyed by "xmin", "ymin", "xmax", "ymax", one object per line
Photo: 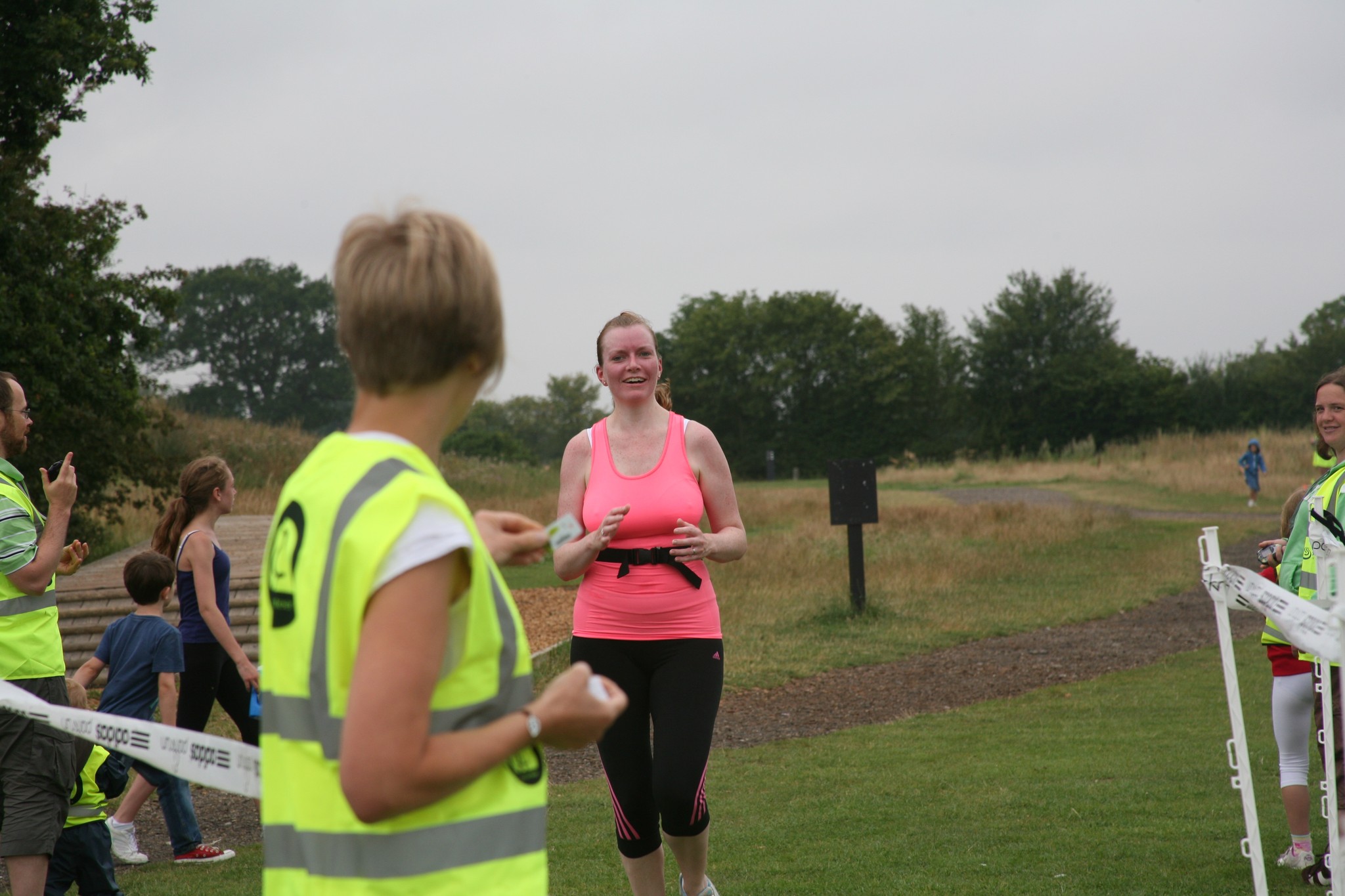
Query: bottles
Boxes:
[{"xmin": 249, "ymin": 665, "xmax": 261, "ymax": 720}]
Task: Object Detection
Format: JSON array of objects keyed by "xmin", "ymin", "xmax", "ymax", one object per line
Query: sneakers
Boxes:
[
  {"xmin": 1303, "ymin": 853, "xmax": 1331, "ymax": 886},
  {"xmin": 103, "ymin": 816, "xmax": 149, "ymax": 864},
  {"xmin": 175, "ymin": 839, "xmax": 235, "ymax": 863},
  {"xmin": 679, "ymin": 871, "xmax": 719, "ymax": 896},
  {"xmin": 1276, "ymin": 845, "xmax": 1315, "ymax": 870}
]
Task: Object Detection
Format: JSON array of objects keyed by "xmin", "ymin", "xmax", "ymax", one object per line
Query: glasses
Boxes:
[{"xmin": 1, "ymin": 408, "xmax": 30, "ymax": 419}]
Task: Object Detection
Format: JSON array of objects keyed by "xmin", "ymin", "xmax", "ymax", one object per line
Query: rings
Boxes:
[
  {"xmin": 691, "ymin": 548, "xmax": 695, "ymax": 556},
  {"xmin": 601, "ymin": 529, "xmax": 607, "ymax": 536}
]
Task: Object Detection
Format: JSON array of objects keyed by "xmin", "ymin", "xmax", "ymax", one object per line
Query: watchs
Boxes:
[{"xmin": 519, "ymin": 709, "xmax": 542, "ymax": 742}]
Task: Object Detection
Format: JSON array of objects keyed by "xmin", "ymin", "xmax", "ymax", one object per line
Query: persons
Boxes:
[
  {"xmin": 1259, "ymin": 366, "xmax": 1345, "ymax": 886},
  {"xmin": 75, "ymin": 549, "xmax": 236, "ymax": 864},
  {"xmin": 106, "ymin": 456, "xmax": 268, "ymax": 863},
  {"xmin": 1238, "ymin": 438, "xmax": 1266, "ymax": 508},
  {"xmin": 554, "ymin": 310, "xmax": 746, "ymax": 896},
  {"xmin": 258, "ymin": 210, "xmax": 627, "ymax": 896},
  {"xmin": 0, "ymin": 374, "xmax": 126, "ymax": 896}
]
